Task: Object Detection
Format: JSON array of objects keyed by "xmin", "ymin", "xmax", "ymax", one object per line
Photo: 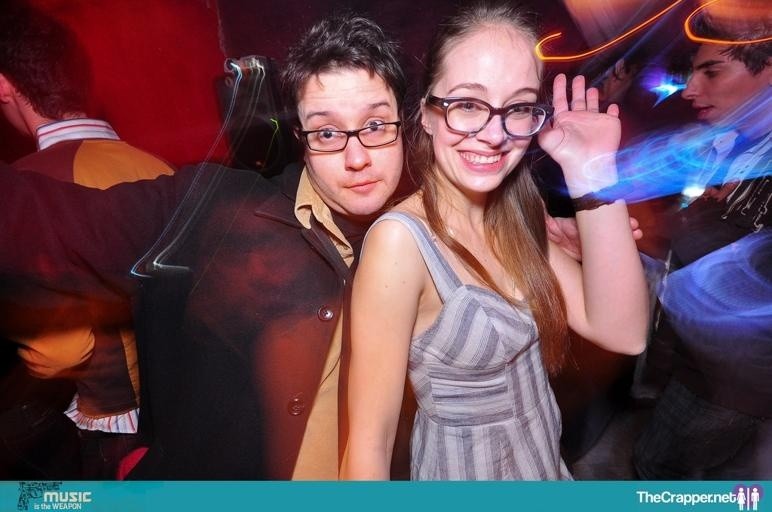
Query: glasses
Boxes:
[
  {"xmin": 290, "ymin": 108, "xmax": 401, "ymax": 152},
  {"xmin": 423, "ymin": 90, "xmax": 554, "ymax": 138}
]
